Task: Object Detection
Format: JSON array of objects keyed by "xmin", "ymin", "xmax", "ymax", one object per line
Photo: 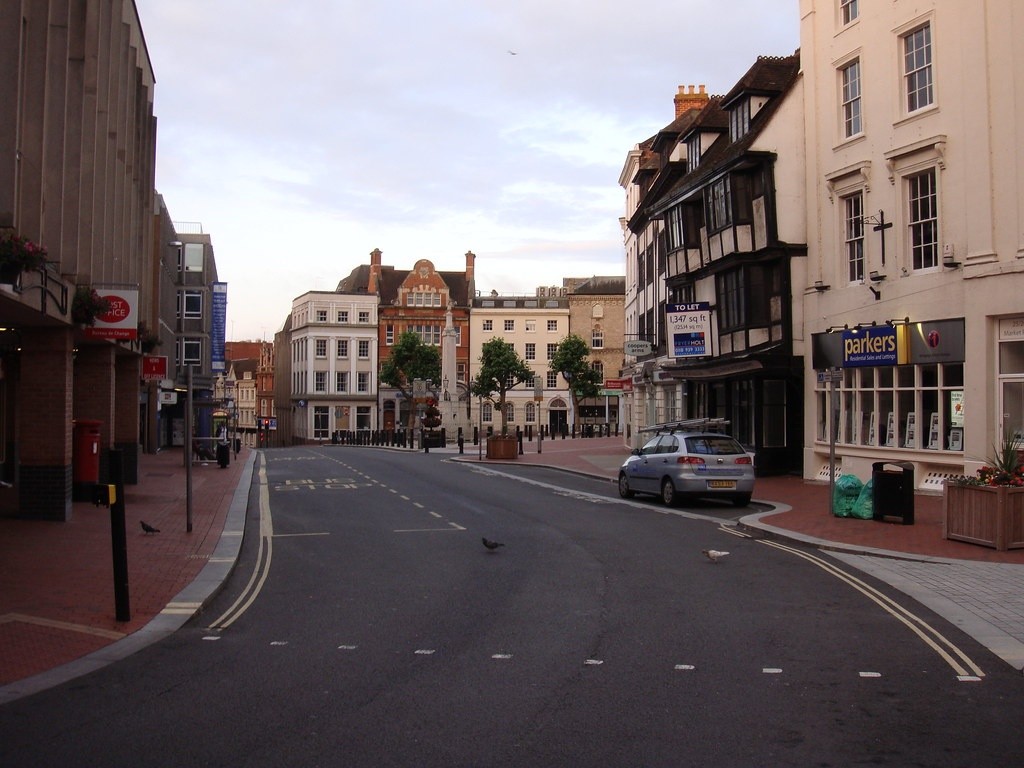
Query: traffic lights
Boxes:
[
  {"xmin": 258, "ymin": 419, "xmax": 262, "ymax": 431},
  {"xmin": 265, "ymin": 419, "xmax": 270, "ymax": 429}
]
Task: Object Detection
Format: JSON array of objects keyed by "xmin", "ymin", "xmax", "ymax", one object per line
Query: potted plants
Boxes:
[
  {"xmin": 418, "ymin": 407, "xmax": 446, "ymax": 449},
  {"xmin": 72, "ymin": 287, "xmax": 111, "ymax": 325},
  {"xmin": 488, "ymin": 432, "xmax": 519, "ymax": 459},
  {"xmin": 141, "ymin": 329, "xmax": 163, "ymax": 353},
  {"xmin": 0, "ymin": 261, "xmax": 20, "ymax": 285}
]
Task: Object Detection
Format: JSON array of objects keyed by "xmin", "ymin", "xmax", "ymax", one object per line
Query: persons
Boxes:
[{"xmin": 195, "ymin": 421, "xmax": 242, "ymax": 468}]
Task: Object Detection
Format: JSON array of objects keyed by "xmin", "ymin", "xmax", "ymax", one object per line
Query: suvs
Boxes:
[{"xmin": 616, "ymin": 423, "xmax": 756, "ymax": 510}]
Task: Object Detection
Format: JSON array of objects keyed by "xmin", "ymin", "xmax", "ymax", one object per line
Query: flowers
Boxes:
[
  {"xmin": 954, "ymin": 429, "xmax": 1024, "ymax": 487},
  {"xmin": 0, "ymin": 230, "xmax": 48, "ymax": 272}
]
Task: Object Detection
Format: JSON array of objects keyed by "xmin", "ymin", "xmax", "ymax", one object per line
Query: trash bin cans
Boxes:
[
  {"xmin": 871, "ymin": 460, "xmax": 915, "ymax": 526},
  {"xmin": 233, "ymin": 437, "xmax": 241, "ymax": 454},
  {"xmin": 216, "ymin": 441, "xmax": 230, "ymax": 468}
]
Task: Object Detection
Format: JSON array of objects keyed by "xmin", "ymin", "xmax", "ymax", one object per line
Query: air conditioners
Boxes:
[
  {"xmin": 536, "ymin": 287, "xmax": 546, "ymax": 297},
  {"xmin": 548, "ymin": 287, "xmax": 557, "ymax": 297},
  {"xmin": 560, "ymin": 287, "xmax": 569, "ymax": 297}
]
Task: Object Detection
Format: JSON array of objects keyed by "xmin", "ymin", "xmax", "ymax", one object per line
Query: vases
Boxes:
[{"xmin": 941, "ymin": 478, "xmax": 1024, "ymax": 550}]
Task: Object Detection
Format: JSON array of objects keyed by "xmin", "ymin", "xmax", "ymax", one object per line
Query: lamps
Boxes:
[
  {"xmin": 869, "ymin": 270, "xmax": 887, "ymax": 281},
  {"xmin": 851, "ymin": 321, "xmax": 876, "ymax": 333},
  {"xmin": 943, "ymin": 256, "xmax": 961, "ymax": 268},
  {"xmin": 885, "ymin": 317, "xmax": 909, "ymax": 328},
  {"xmin": 815, "ymin": 280, "xmax": 830, "ymax": 290},
  {"xmin": 826, "ymin": 323, "xmax": 848, "ymax": 334}
]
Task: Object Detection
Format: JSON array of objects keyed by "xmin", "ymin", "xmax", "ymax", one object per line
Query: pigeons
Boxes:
[
  {"xmin": 482, "ymin": 537, "xmax": 504, "ymax": 553},
  {"xmin": 702, "ymin": 549, "xmax": 730, "ymax": 563},
  {"xmin": 140, "ymin": 520, "xmax": 160, "ymax": 536}
]
